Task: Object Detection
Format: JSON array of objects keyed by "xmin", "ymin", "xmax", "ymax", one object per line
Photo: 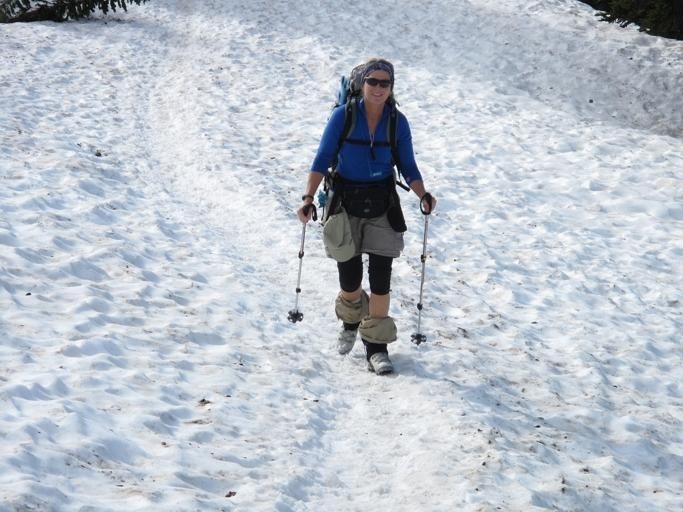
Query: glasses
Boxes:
[{"xmin": 365, "ymin": 77, "xmax": 391, "ymax": 88}]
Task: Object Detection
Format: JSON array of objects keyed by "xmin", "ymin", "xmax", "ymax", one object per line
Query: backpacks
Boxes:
[{"xmin": 329, "ymin": 73, "xmax": 398, "ymax": 159}]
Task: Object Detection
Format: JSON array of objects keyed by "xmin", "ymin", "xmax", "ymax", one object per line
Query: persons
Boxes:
[{"xmin": 297, "ymin": 58, "xmax": 436, "ymax": 376}]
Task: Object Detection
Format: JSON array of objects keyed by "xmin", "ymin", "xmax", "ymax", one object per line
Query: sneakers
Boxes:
[
  {"xmin": 367, "ymin": 352, "xmax": 393, "ymax": 375},
  {"xmin": 336, "ymin": 326, "xmax": 357, "ymax": 355}
]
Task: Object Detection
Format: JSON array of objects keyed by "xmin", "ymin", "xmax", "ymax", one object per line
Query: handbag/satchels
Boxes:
[{"xmin": 341, "ymin": 185, "xmax": 391, "ymax": 219}]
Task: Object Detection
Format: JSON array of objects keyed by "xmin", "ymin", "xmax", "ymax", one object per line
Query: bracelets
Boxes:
[{"xmin": 300, "ymin": 194, "xmax": 312, "ymax": 200}]
